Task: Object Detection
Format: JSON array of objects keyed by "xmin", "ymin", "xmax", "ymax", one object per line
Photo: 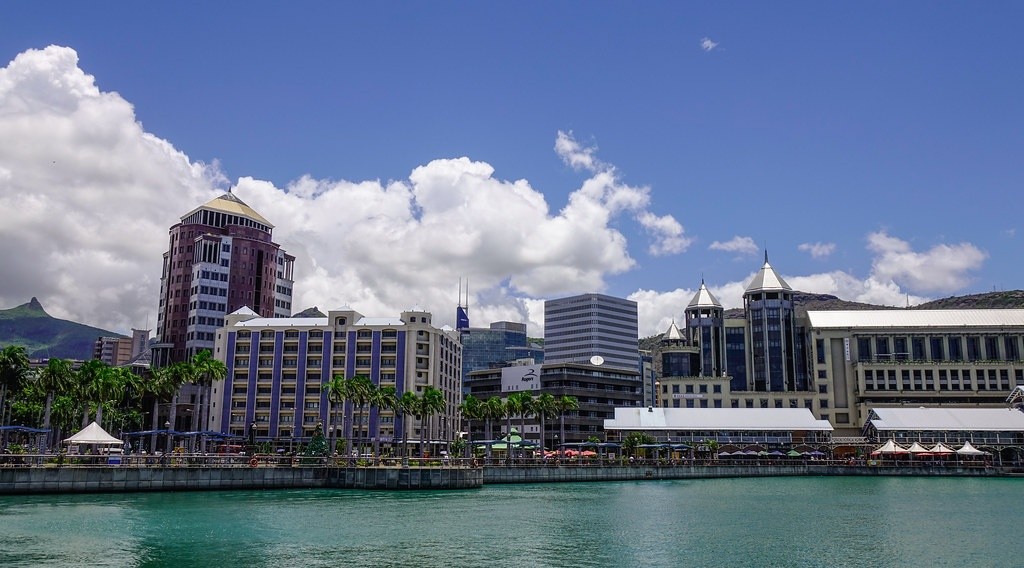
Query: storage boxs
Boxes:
[{"xmin": 108, "ymin": 456, "xmax": 121, "ymax": 464}]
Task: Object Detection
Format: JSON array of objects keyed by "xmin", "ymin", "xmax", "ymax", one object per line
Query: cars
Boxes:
[{"xmin": 1, "ymin": 442, "xmax": 51, "ymax": 455}]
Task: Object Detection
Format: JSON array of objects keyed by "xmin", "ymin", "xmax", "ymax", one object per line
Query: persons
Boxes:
[
  {"xmin": 159, "ymin": 452, "xmax": 166, "ymax": 464},
  {"xmin": 350, "ymin": 451, "xmax": 356, "ymax": 467},
  {"xmin": 95, "ymin": 450, "xmax": 105, "ymax": 464},
  {"xmin": 16, "ymin": 434, "xmax": 27, "ymax": 444},
  {"xmin": 505, "ymin": 456, "xmax": 512, "ymax": 466},
  {"xmin": 639, "ymin": 455, "xmax": 643, "ymax": 465},
  {"xmin": 470, "ymin": 459, "xmax": 478, "ymax": 468},
  {"xmin": 334, "ymin": 450, "xmax": 338, "ymax": 457},
  {"xmin": 382, "ymin": 452, "xmax": 387, "ymax": 464},
  {"xmin": 537, "ymin": 454, "xmax": 546, "ymax": 464},
  {"xmin": 146, "ymin": 453, "xmax": 154, "ymax": 464},
  {"xmin": 682, "ymin": 456, "xmax": 685, "ymax": 465},
  {"xmin": 176, "ymin": 448, "xmax": 181, "ymax": 467},
  {"xmin": 554, "ymin": 455, "xmax": 560, "ymax": 466},
  {"xmin": 630, "ymin": 455, "xmax": 635, "ymax": 463},
  {"xmin": 319, "ymin": 449, "xmax": 327, "ymax": 464}
]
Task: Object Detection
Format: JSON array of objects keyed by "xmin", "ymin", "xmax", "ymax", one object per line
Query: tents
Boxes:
[
  {"xmin": 876, "ymin": 440, "xmax": 984, "ymax": 453},
  {"xmin": 63, "ymin": 421, "xmax": 124, "ymax": 455}
]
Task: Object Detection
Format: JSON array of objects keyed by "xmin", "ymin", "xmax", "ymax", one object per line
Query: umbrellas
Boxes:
[
  {"xmin": 719, "ymin": 450, "xmax": 825, "ymax": 457},
  {"xmin": 537, "ymin": 449, "xmax": 596, "ymax": 455}
]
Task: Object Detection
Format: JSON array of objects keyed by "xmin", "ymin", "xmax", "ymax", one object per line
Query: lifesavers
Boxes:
[
  {"xmin": 250, "ymin": 458, "xmax": 258, "ymax": 468},
  {"xmin": 474, "ymin": 459, "xmax": 479, "ymax": 467}
]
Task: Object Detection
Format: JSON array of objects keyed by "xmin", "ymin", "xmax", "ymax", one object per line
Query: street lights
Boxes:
[
  {"xmin": 251, "ymin": 423, "xmax": 257, "ymax": 455},
  {"xmin": 164, "ymin": 420, "xmax": 171, "ymax": 452},
  {"xmin": 290, "ymin": 408, "xmax": 297, "ymax": 437}
]
